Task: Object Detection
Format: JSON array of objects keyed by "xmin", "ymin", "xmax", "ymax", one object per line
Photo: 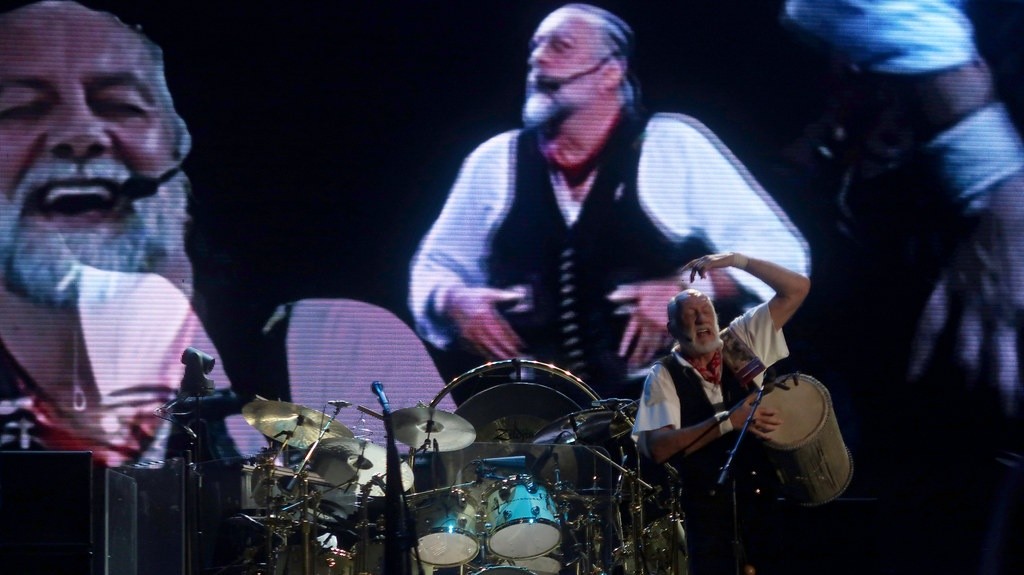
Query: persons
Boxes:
[
  {"xmin": 630, "ymin": 251, "xmax": 812, "ymax": 575},
  {"xmin": 0, "ymin": 0, "xmax": 460, "ymax": 508},
  {"xmin": 407, "ymin": 4, "xmax": 809, "ymax": 411},
  {"xmin": 770, "ymin": 0, "xmax": 1024, "ymax": 435}
]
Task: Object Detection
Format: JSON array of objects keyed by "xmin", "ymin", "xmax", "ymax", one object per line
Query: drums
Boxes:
[
  {"xmin": 478, "ymin": 471, "xmax": 565, "ymax": 563},
  {"xmin": 466, "ymin": 562, "xmax": 541, "ymax": 575},
  {"xmin": 746, "ymin": 367, "xmax": 857, "ymax": 511},
  {"xmin": 406, "ymin": 487, "xmax": 483, "ymax": 569}
]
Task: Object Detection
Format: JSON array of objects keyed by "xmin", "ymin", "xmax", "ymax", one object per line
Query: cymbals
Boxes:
[
  {"xmin": 383, "ymin": 405, "xmax": 478, "ymax": 453},
  {"xmin": 306, "ymin": 436, "xmax": 416, "ymax": 498},
  {"xmin": 532, "ymin": 407, "xmax": 616, "ymax": 446},
  {"xmin": 240, "ymin": 399, "xmax": 356, "ymax": 452}
]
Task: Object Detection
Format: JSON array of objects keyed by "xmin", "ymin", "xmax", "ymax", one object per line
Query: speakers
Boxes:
[{"xmin": 0, "ymin": 450, "xmax": 95, "ymax": 575}]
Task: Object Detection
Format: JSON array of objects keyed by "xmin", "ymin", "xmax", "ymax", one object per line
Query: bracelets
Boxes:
[
  {"xmin": 679, "ymin": 269, "xmax": 715, "ymax": 302},
  {"xmin": 714, "ymin": 411, "xmax": 733, "ymax": 434},
  {"xmin": 732, "ymin": 253, "xmax": 748, "ymax": 270},
  {"xmin": 435, "ymin": 286, "xmax": 454, "ymax": 318}
]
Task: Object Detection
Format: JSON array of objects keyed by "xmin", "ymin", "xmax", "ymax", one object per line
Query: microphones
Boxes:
[
  {"xmin": 529, "ymin": 432, "xmax": 565, "ymax": 473},
  {"xmin": 592, "ymin": 398, "xmax": 633, "ymax": 407},
  {"xmin": 121, "ymin": 167, "xmax": 183, "ymax": 199},
  {"xmin": 683, "ymin": 335, "xmax": 693, "ymax": 342},
  {"xmin": 534, "ymin": 53, "xmax": 616, "ymax": 93},
  {"xmin": 432, "ymin": 438, "xmax": 447, "ymax": 487},
  {"xmin": 242, "ymin": 514, "xmax": 285, "ymax": 545},
  {"xmin": 328, "ymin": 400, "xmax": 353, "ymax": 407},
  {"xmin": 766, "ymin": 365, "xmax": 776, "ymax": 383},
  {"xmin": 371, "ymin": 381, "xmax": 391, "ymax": 411}
]
